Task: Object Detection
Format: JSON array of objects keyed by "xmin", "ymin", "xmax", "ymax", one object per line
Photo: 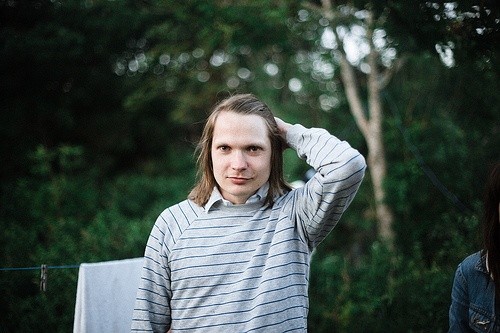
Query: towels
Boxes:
[{"xmin": 72, "ymin": 257, "xmax": 144, "ymax": 332}]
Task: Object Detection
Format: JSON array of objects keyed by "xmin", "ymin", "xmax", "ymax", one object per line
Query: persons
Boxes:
[
  {"xmin": 448, "ymin": 162, "xmax": 500, "ymax": 331},
  {"xmin": 131, "ymin": 93, "xmax": 367, "ymax": 333}
]
